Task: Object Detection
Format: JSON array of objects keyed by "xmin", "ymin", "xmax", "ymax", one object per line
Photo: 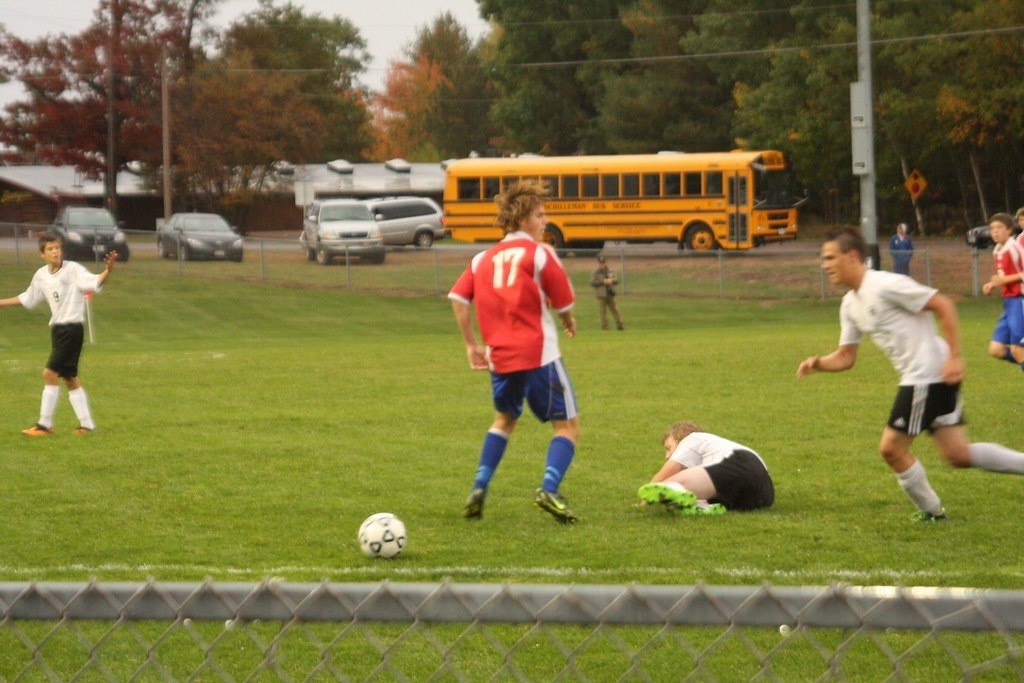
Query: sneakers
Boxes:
[
  {"xmin": 463, "ymin": 488, "xmax": 482, "ymax": 520},
  {"xmin": 535, "ymin": 487, "xmax": 577, "ymax": 524},
  {"xmin": 672, "ymin": 499, "xmax": 726, "ymax": 517},
  {"xmin": 637, "ymin": 481, "xmax": 696, "ymax": 507}
]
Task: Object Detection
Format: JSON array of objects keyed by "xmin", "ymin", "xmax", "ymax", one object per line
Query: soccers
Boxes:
[{"xmin": 356, "ymin": 511, "xmax": 408, "ymax": 559}]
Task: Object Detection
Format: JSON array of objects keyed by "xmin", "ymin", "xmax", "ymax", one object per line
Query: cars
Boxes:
[
  {"xmin": 156, "ymin": 212, "xmax": 245, "ymax": 263},
  {"xmin": 966, "ymin": 214, "xmax": 1023, "ymax": 250}
]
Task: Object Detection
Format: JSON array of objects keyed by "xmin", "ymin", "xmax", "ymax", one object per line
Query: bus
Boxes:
[{"xmin": 442, "ymin": 145, "xmax": 811, "ymax": 256}]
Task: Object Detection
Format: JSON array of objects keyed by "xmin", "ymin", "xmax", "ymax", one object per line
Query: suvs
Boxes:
[
  {"xmin": 50, "ymin": 204, "xmax": 130, "ymax": 262},
  {"xmin": 364, "ymin": 195, "xmax": 449, "ymax": 251},
  {"xmin": 304, "ymin": 196, "xmax": 386, "ymax": 266}
]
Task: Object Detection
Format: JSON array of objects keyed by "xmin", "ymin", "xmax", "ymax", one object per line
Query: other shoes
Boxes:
[
  {"xmin": 912, "ymin": 506, "xmax": 946, "ymax": 523},
  {"xmin": 22, "ymin": 423, "xmax": 53, "ymax": 436},
  {"xmin": 73, "ymin": 426, "xmax": 95, "ymax": 435}
]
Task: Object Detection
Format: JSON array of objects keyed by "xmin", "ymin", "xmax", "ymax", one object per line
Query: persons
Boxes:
[
  {"xmin": 590, "ymin": 255, "xmax": 624, "ymax": 330},
  {"xmin": 795, "ymin": 226, "xmax": 1024, "ymax": 523},
  {"xmin": 983, "ymin": 207, "xmax": 1024, "ymax": 372},
  {"xmin": 632, "ymin": 420, "xmax": 775, "ymax": 516},
  {"xmin": 0, "ymin": 234, "xmax": 117, "ymax": 436},
  {"xmin": 448, "ymin": 181, "xmax": 583, "ymax": 523},
  {"xmin": 889, "ymin": 223, "xmax": 914, "ymax": 276}
]
